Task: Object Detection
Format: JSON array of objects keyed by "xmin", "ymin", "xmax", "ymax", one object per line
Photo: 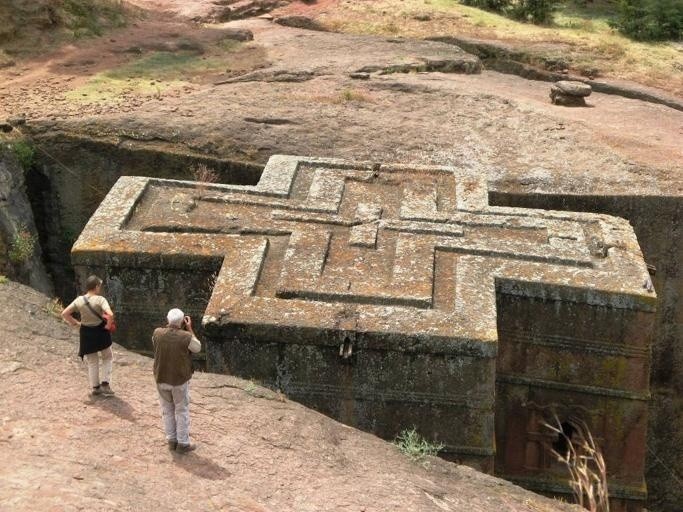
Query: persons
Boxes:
[
  {"xmin": 58, "ymin": 275, "xmax": 114, "ymax": 395},
  {"xmin": 150, "ymin": 308, "xmax": 201, "ymax": 453}
]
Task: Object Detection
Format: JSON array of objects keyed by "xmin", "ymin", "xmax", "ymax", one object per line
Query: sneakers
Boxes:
[
  {"xmin": 90, "ymin": 381, "xmax": 115, "ymax": 397},
  {"xmin": 168, "ymin": 438, "xmax": 197, "ymax": 454}
]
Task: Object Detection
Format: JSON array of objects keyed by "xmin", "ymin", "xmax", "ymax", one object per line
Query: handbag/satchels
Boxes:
[{"xmin": 103, "ymin": 312, "xmax": 117, "ymax": 334}]
